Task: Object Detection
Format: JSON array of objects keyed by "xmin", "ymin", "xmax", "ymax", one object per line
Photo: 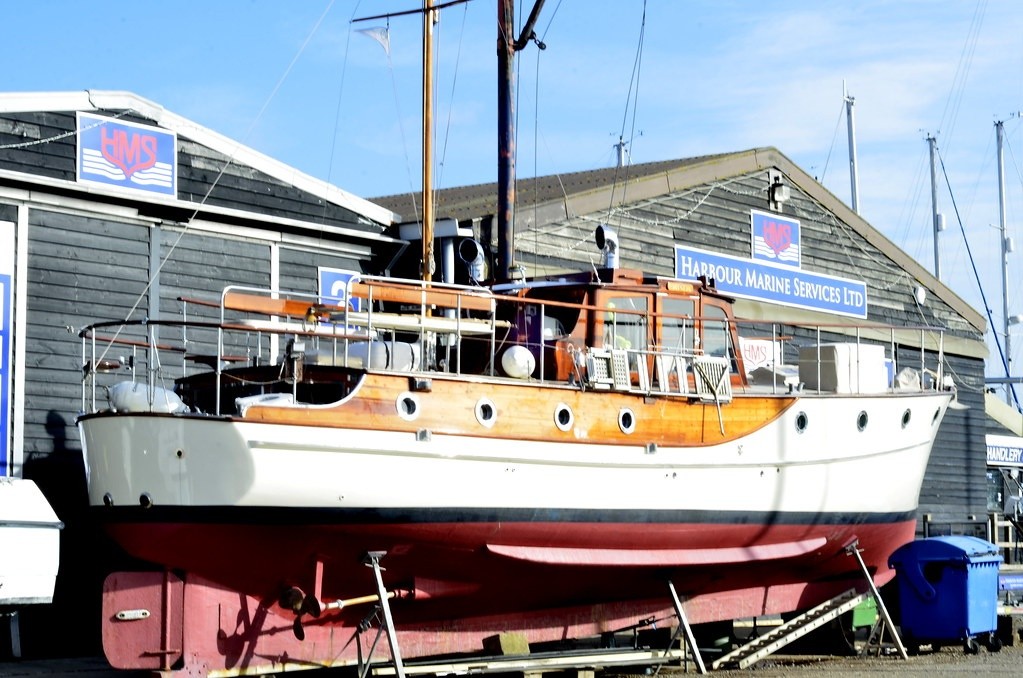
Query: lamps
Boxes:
[{"xmin": 772, "ymin": 176, "xmax": 790, "ymax": 209}]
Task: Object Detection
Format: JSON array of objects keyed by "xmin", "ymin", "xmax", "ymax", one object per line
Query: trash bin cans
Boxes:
[{"xmin": 888, "ymin": 535, "xmax": 1004, "ymax": 654}]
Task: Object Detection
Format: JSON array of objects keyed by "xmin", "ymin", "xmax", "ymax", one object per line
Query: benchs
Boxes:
[
  {"xmin": 331, "ymin": 276, "xmax": 497, "ymax": 371},
  {"xmin": 219, "ymin": 288, "xmax": 378, "ymax": 371}
]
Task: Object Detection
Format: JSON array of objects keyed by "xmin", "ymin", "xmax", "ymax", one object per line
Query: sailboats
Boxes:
[{"xmin": 71, "ymin": 0, "xmax": 962, "ymax": 678}]
